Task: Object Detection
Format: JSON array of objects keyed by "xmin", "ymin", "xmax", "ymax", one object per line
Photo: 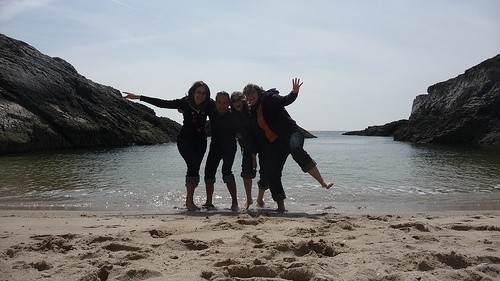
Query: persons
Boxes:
[
  {"xmin": 122, "ymin": 81, "xmax": 210, "ymax": 212},
  {"xmin": 202, "ymin": 88, "xmax": 279, "ymax": 210},
  {"xmin": 243, "ymin": 78, "xmax": 333, "ymax": 211}
]
